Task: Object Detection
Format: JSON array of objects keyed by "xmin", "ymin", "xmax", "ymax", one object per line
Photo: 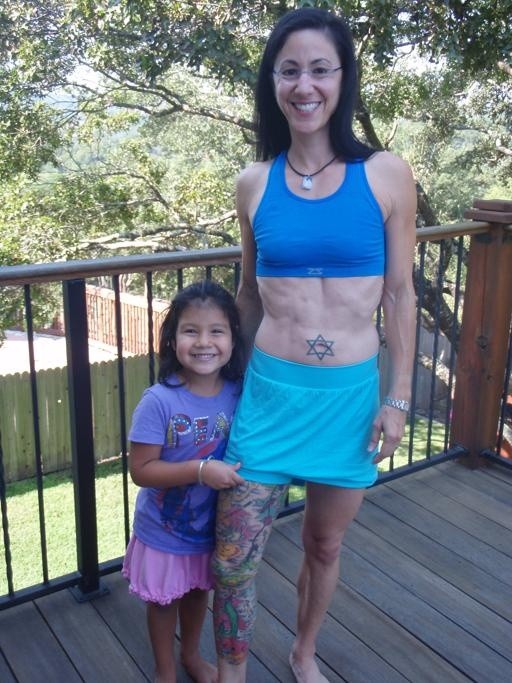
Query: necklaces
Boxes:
[{"xmin": 285, "ymin": 152, "xmax": 338, "ymax": 190}]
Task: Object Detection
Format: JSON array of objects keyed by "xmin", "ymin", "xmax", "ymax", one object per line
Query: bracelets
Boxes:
[
  {"xmin": 198, "ymin": 455, "xmax": 214, "ymax": 487},
  {"xmin": 382, "ymin": 397, "xmax": 410, "ymax": 413}
]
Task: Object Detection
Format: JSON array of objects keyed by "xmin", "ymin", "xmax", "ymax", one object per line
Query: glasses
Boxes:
[{"xmin": 271, "ymin": 65, "xmax": 345, "ymax": 81}]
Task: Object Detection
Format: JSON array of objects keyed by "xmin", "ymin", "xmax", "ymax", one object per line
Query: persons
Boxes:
[
  {"xmin": 121, "ymin": 279, "xmax": 251, "ymax": 683},
  {"xmin": 206, "ymin": 5, "xmax": 420, "ymax": 683}
]
